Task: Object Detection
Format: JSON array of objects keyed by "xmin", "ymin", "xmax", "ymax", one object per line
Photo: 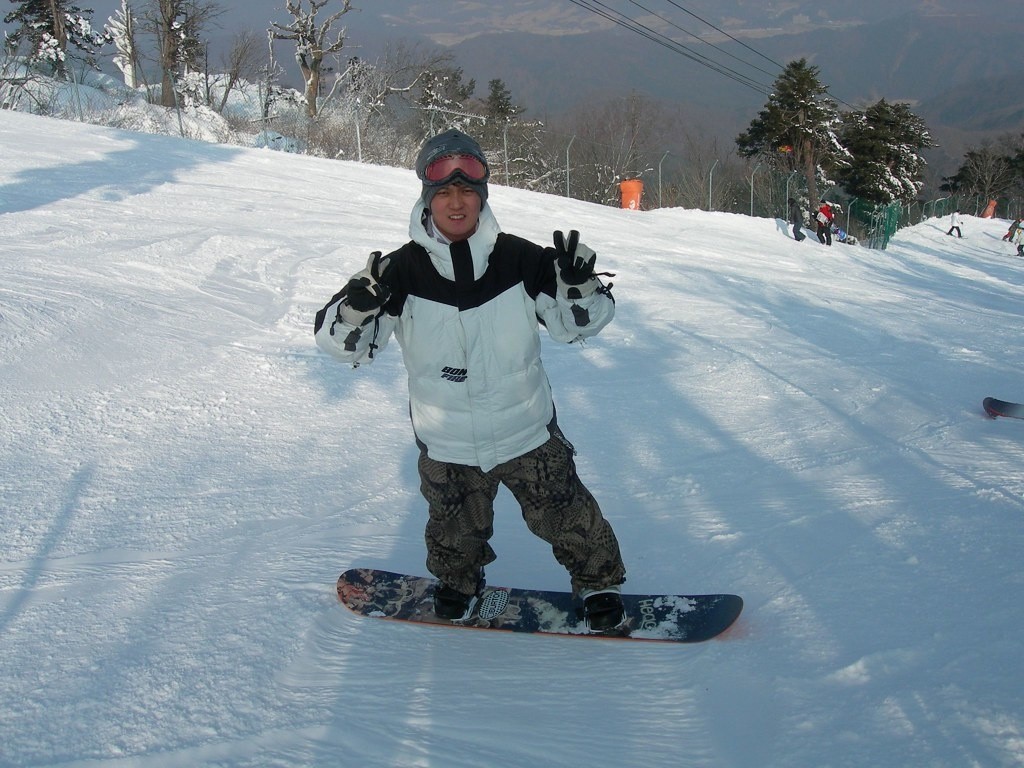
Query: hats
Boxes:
[{"xmin": 414, "ymin": 130, "xmax": 489, "ymax": 212}]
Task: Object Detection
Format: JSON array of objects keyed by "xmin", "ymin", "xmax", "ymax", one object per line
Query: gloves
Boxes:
[
  {"xmin": 347, "ymin": 250, "xmax": 393, "ymax": 313},
  {"xmin": 544, "ymin": 230, "xmax": 596, "ymax": 285}
]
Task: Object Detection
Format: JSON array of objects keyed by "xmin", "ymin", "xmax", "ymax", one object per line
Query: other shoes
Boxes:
[
  {"xmin": 434, "ymin": 584, "xmax": 480, "ymax": 622},
  {"xmin": 581, "ymin": 589, "xmax": 623, "ymax": 630}
]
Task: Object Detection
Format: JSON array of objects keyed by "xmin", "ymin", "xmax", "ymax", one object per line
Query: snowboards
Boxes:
[
  {"xmin": 984, "ymin": 396, "xmax": 1024, "ymax": 420},
  {"xmin": 338, "ymin": 567, "xmax": 744, "ymax": 642}
]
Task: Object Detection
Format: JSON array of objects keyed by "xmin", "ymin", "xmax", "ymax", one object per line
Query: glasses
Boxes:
[{"xmin": 421, "ymin": 153, "xmax": 490, "ymax": 187}]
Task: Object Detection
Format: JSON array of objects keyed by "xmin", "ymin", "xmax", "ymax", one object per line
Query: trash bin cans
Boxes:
[
  {"xmin": 982, "ymin": 200, "xmax": 997, "ymax": 220},
  {"xmin": 620, "ymin": 178, "xmax": 643, "ymax": 212}
]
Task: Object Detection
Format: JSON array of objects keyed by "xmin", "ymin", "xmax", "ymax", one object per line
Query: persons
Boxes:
[
  {"xmin": 788, "ymin": 197, "xmax": 807, "ymax": 241},
  {"xmin": 811, "ymin": 199, "xmax": 835, "ymax": 245},
  {"xmin": 946, "ymin": 209, "xmax": 964, "ymax": 238},
  {"xmin": 313, "ymin": 126, "xmax": 628, "ymax": 632},
  {"xmin": 1001, "ymin": 218, "xmax": 1024, "ymax": 257}
]
